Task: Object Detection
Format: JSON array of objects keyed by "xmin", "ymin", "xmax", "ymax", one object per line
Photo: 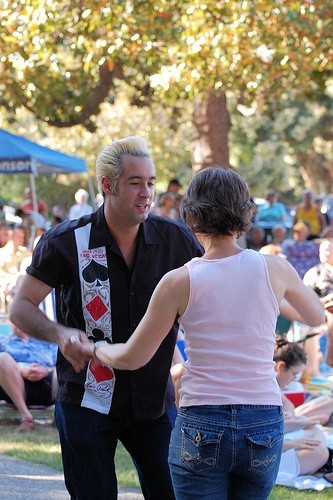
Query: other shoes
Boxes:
[{"xmin": 16, "ymin": 417, "xmax": 34, "ymax": 432}]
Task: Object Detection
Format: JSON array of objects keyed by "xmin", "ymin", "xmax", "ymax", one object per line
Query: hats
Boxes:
[{"xmin": 293, "ymin": 222, "xmax": 309, "ymax": 233}]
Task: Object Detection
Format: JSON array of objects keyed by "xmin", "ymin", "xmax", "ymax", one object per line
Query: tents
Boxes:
[{"xmin": 0, "ymin": 128, "xmax": 86, "ymax": 212}]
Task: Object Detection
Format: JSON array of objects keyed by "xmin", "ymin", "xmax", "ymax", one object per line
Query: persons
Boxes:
[
  {"xmin": 273, "ymin": 239, "xmax": 333, "ymax": 491},
  {"xmin": 0, "ymin": 187, "xmax": 104, "ymax": 315},
  {"xmin": 0, "ymin": 325, "xmax": 56, "ymax": 433},
  {"xmin": 10, "ymin": 138, "xmax": 205, "ymax": 500},
  {"xmin": 81, "ymin": 167, "xmax": 325, "ymax": 500},
  {"xmin": 153, "ymin": 178, "xmax": 333, "ymax": 281}
]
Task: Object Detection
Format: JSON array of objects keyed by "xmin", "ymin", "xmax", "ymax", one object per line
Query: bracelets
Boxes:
[{"xmin": 94, "ymin": 341, "xmax": 107, "ymax": 353}]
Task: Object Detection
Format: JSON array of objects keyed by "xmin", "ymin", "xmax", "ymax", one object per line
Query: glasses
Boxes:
[{"xmin": 289, "ymin": 367, "xmax": 301, "ymax": 380}]
